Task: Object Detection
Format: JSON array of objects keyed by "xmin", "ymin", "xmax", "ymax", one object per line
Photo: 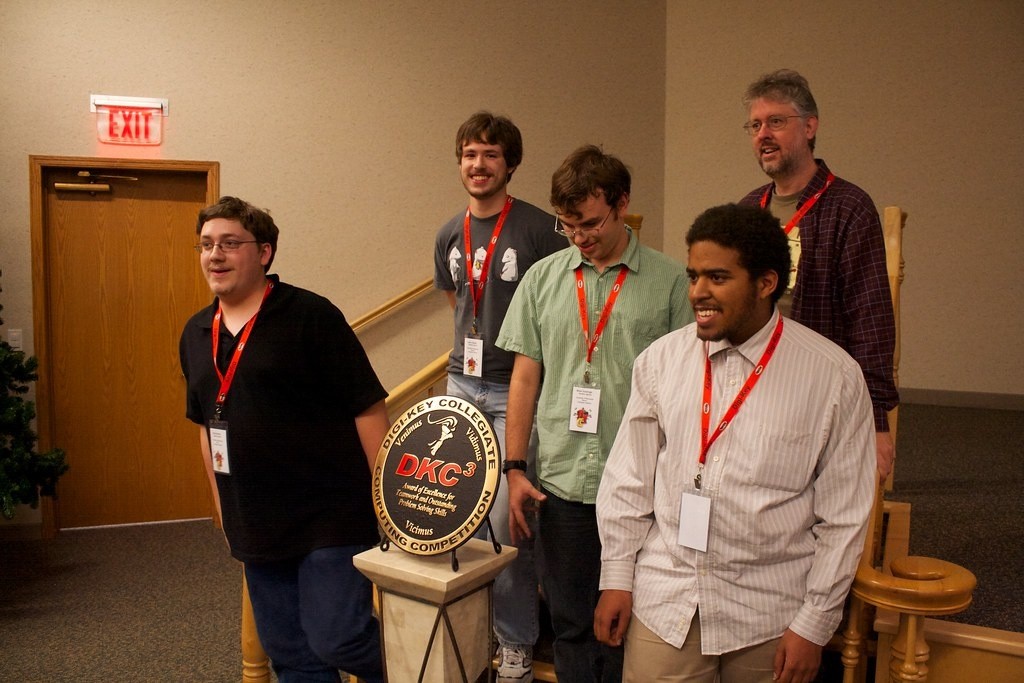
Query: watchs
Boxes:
[{"xmin": 502, "ymin": 459, "xmax": 527, "ymax": 474}]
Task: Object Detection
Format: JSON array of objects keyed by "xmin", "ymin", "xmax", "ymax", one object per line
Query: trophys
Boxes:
[{"xmin": 371, "ymin": 395, "xmax": 503, "ymax": 573}]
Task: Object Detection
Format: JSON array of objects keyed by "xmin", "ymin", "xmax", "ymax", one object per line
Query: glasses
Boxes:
[
  {"xmin": 555, "ymin": 198, "xmax": 619, "ymax": 238},
  {"xmin": 742, "ymin": 114, "xmax": 807, "ymax": 137},
  {"xmin": 193, "ymin": 240, "xmax": 260, "ymax": 253}
]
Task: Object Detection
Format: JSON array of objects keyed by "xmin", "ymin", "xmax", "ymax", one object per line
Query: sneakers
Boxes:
[{"xmin": 496, "ymin": 645, "xmax": 534, "ymax": 683}]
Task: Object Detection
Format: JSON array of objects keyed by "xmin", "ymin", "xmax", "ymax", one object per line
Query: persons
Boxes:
[
  {"xmin": 593, "ymin": 202, "xmax": 876, "ymax": 683},
  {"xmin": 177, "ymin": 196, "xmax": 391, "ymax": 683},
  {"xmin": 434, "ymin": 111, "xmax": 571, "ymax": 683},
  {"xmin": 494, "ymin": 146, "xmax": 695, "ymax": 683},
  {"xmin": 736, "ymin": 66, "xmax": 900, "ymax": 486}
]
